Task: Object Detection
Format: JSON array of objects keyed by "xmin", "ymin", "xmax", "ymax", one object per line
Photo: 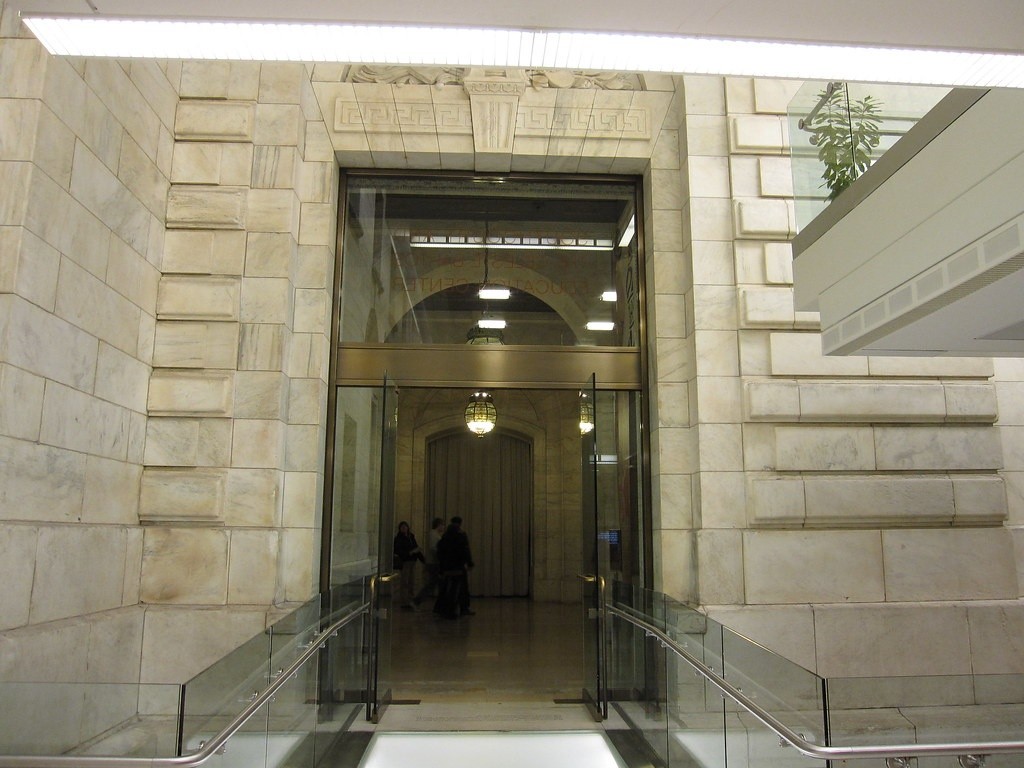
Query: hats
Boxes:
[{"xmin": 451, "ymin": 516, "xmax": 462, "ymax": 523}]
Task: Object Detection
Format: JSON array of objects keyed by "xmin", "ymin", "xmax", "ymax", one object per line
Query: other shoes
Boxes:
[
  {"xmin": 401, "ymin": 604, "xmax": 410, "ymax": 608},
  {"xmin": 433, "ymin": 607, "xmax": 475, "ymax": 619},
  {"xmin": 409, "ymin": 602, "xmax": 419, "ymax": 608}
]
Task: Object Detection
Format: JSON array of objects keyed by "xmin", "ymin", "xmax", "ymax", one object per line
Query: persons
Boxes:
[{"xmin": 392, "ymin": 516, "xmax": 475, "ymax": 618}]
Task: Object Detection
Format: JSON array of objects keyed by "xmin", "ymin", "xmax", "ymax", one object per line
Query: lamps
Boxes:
[
  {"xmin": 465, "ymin": 326, "xmax": 505, "ymax": 345},
  {"xmin": 464, "ymin": 390, "xmax": 498, "ymax": 438},
  {"xmin": 580, "ymin": 401, "xmax": 594, "ymax": 435}
]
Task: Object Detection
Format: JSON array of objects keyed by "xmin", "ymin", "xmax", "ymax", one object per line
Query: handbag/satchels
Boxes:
[{"xmin": 393, "ymin": 556, "xmax": 403, "ymax": 570}]
